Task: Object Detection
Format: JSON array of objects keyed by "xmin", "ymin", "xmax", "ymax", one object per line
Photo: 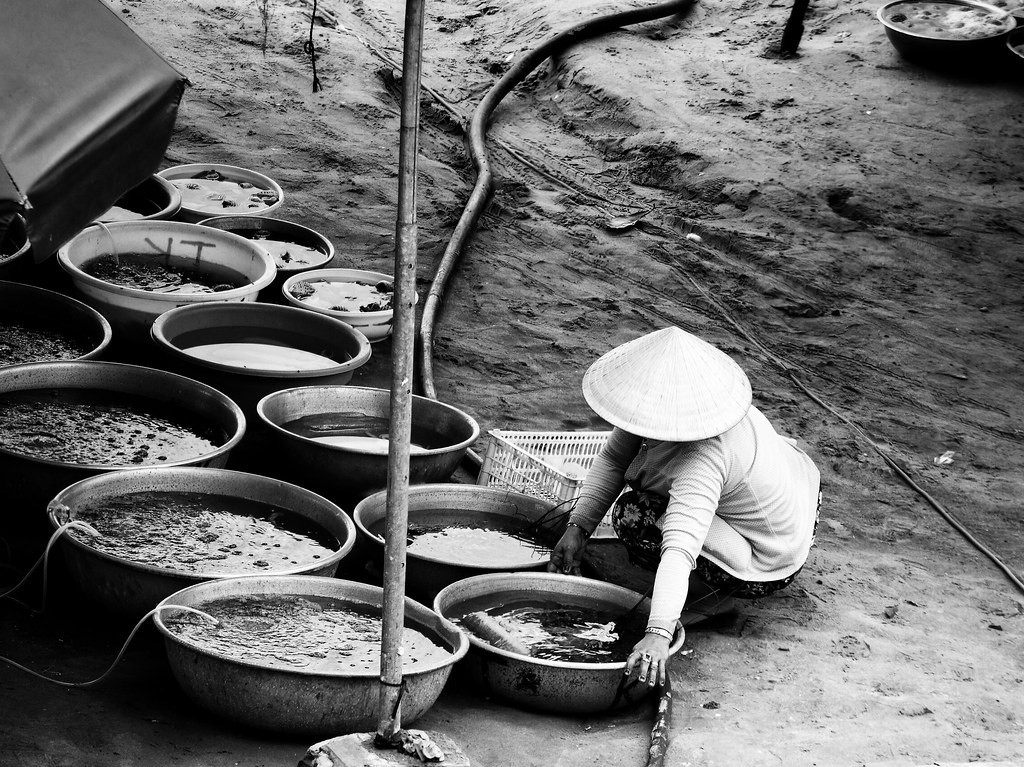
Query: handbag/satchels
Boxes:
[{"xmin": 0, "ymin": 0, "xmax": 191, "ymax": 263}]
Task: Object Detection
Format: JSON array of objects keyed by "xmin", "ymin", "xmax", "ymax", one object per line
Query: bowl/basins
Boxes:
[
  {"xmin": 0, "ymin": 209, "xmax": 32, "ymax": 266},
  {"xmin": 152, "ymin": 574, "xmax": 470, "ymax": 742},
  {"xmin": 877, "ymin": 0, "xmax": 1017, "ymax": 60},
  {"xmin": 1004, "ymin": 25, "xmax": 1024, "ymax": 63},
  {"xmin": 50, "ymin": 465, "xmax": 357, "ymax": 613},
  {"xmin": 352, "ymin": 481, "xmax": 575, "ymax": 593},
  {"xmin": 432, "ymin": 570, "xmax": 686, "ymax": 712},
  {"xmin": 56, "ymin": 220, "xmax": 278, "ymax": 334},
  {"xmin": 157, "ymin": 163, "xmax": 285, "ymax": 224},
  {"xmin": 149, "ymin": 300, "xmax": 373, "ymax": 415},
  {"xmin": 195, "ymin": 215, "xmax": 335, "ymax": 288},
  {"xmin": 256, "ymin": 384, "xmax": 481, "ymax": 488},
  {"xmin": 85, "ymin": 173, "xmax": 183, "ymax": 225},
  {"xmin": 0, "ymin": 359, "xmax": 247, "ymax": 493},
  {"xmin": 0, "ymin": 279, "xmax": 112, "ymax": 367},
  {"xmin": 281, "ymin": 268, "xmax": 419, "ymax": 343}
]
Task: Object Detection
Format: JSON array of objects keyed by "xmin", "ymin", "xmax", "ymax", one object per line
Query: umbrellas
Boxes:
[{"xmin": 0, "ymin": 0, "xmax": 187, "ymax": 262}]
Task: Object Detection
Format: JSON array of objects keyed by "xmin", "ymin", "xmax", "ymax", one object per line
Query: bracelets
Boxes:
[
  {"xmin": 644, "ymin": 628, "xmax": 674, "ymax": 642},
  {"xmin": 566, "ymin": 522, "xmax": 589, "ymax": 542}
]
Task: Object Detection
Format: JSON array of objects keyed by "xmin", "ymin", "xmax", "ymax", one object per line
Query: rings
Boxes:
[
  {"xmin": 651, "ymin": 662, "xmax": 658, "ymax": 666},
  {"xmin": 641, "ymin": 653, "xmax": 650, "ymax": 664}
]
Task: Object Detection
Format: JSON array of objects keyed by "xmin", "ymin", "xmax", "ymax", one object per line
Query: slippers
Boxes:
[{"xmin": 680, "ymin": 607, "xmax": 735, "ymax": 629}]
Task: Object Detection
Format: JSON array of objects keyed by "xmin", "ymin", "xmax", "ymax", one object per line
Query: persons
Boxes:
[{"xmin": 546, "ymin": 326, "xmax": 824, "ymax": 685}]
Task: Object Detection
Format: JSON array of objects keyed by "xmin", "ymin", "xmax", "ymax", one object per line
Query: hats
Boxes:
[{"xmin": 583, "ymin": 326, "xmax": 753, "ymax": 441}]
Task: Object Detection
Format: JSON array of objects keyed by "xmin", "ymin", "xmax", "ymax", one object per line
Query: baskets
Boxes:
[{"xmin": 476, "ymin": 430, "xmax": 633, "ymax": 539}]
